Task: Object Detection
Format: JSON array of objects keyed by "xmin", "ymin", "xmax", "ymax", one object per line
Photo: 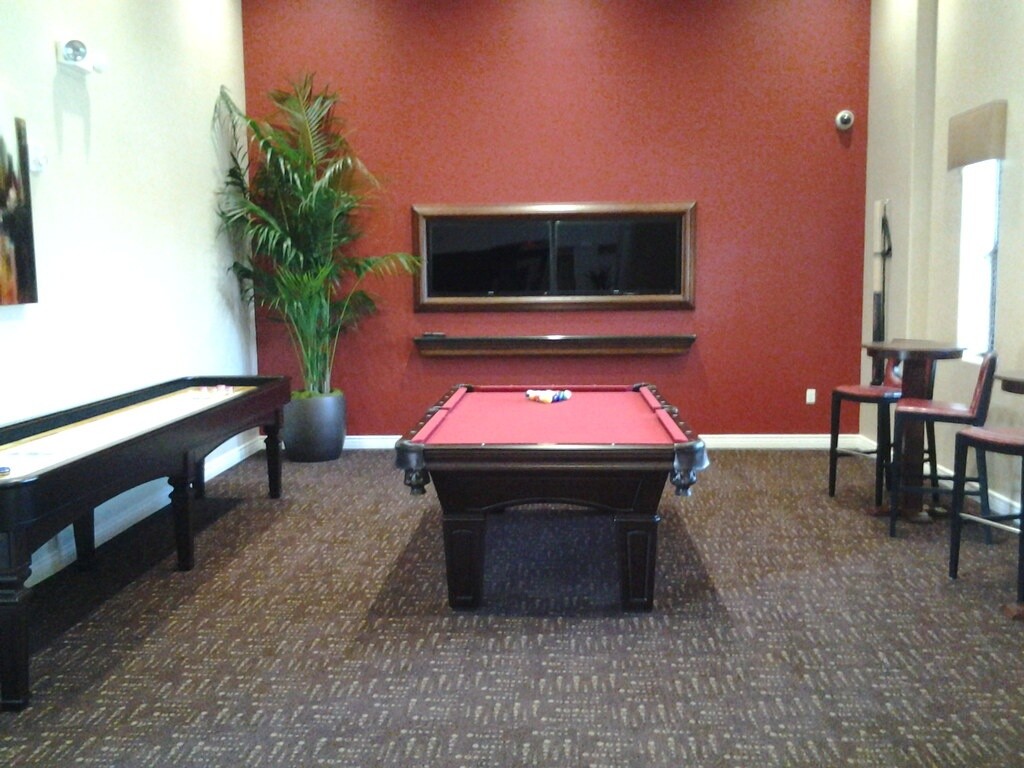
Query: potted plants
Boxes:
[{"xmin": 212, "ymin": 71, "xmax": 427, "ymax": 462}]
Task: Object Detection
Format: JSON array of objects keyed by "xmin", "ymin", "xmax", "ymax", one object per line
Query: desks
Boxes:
[
  {"xmin": 394, "ymin": 382, "xmax": 711, "ymax": 614},
  {"xmin": 867, "ymin": 338, "xmax": 965, "ymax": 522},
  {"xmin": 0, "ymin": 372, "xmax": 293, "ymax": 708}
]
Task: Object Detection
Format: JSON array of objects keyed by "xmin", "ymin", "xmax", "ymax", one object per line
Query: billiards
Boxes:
[{"xmin": 526, "ymin": 389, "xmax": 572, "ymax": 404}]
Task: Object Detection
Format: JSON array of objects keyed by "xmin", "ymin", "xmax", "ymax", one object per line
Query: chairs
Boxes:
[{"xmin": 830, "ymin": 352, "xmax": 1024, "ymax": 604}]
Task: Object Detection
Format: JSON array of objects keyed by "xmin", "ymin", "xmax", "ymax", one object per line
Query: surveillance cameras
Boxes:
[{"xmin": 834, "ymin": 109, "xmax": 855, "ymax": 130}]
